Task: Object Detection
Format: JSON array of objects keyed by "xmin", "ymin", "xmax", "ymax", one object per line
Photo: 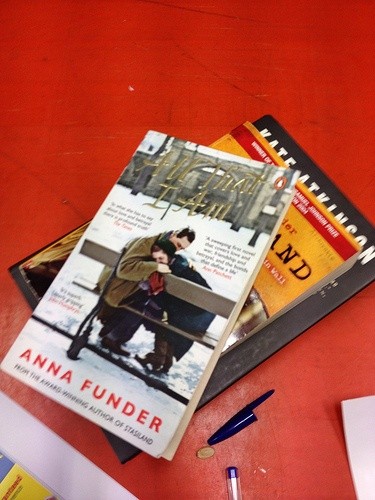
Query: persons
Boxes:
[
  {"xmin": 97, "ymin": 227, "xmax": 197, "ymax": 358},
  {"xmin": 137, "ymin": 238, "xmax": 216, "ymax": 372}
]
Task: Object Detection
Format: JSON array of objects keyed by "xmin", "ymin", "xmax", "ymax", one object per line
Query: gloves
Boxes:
[{"xmin": 149, "ymin": 272, "xmax": 164, "ymax": 294}]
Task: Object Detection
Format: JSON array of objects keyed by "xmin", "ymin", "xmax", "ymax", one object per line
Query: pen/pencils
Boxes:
[{"xmin": 227, "ymin": 466, "xmax": 242, "ymax": 500}]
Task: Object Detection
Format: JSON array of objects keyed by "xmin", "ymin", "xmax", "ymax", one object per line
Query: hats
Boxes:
[{"xmin": 156, "ymin": 239, "xmax": 176, "ymax": 256}]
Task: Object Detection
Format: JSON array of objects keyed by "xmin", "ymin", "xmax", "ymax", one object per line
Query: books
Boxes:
[
  {"xmin": 1, "ymin": 127, "xmax": 302, "ymax": 466},
  {"xmin": 6, "ymin": 110, "xmax": 375, "ymax": 467},
  {"xmin": 18, "ymin": 121, "xmax": 364, "ymax": 364}
]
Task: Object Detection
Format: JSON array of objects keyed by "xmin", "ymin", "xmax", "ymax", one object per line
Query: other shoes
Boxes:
[
  {"xmin": 135, "ymin": 352, "xmax": 171, "ymax": 375},
  {"xmin": 101, "ymin": 336, "xmax": 130, "ymax": 357}
]
